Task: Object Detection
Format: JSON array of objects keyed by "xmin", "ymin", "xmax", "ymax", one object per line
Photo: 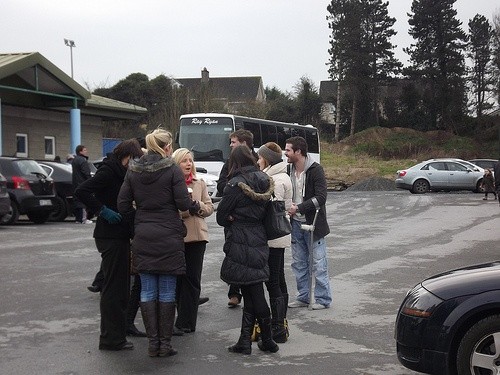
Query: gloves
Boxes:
[{"xmin": 100, "ymin": 207, "xmax": 122, "ymax": 224}]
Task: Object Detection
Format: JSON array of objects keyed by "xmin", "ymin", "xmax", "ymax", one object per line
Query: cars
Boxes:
[
  {"xmin": 469, "ymin": 158, "xmax": 500, "ymax": 172},
  {"xmin": 393, "ymin": 258, "xmax": 500, "ymax": 375},
  {"xmin": 0, "ymin": 156, "xmax": 103, "ymax": 226},
  {"xmin": 394, "ymin": 157, "xmax": 496, "ymax": 194}
]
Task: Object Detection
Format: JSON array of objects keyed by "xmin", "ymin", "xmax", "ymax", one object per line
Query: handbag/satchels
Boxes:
[{"xmin": 263, "ymin": 200, "xmax": 292, "ymax": 241}]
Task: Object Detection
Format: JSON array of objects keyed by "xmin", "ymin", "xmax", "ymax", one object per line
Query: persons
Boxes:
[
  {"xmin": 257, "ymin": 141, "xmax": 293, "ymax": 343},
  {"xmin": 217, "ymin": 129, "xmax": 259, "ymax": 306},
  {"xmin": 286, "ymin": 137, "xmax": 333, "ymax": 310},
  {"xmin": 215, "ymin": 144, "xmax": 280, "ymax": 354},
  {"xmin": 66, "ymin": 145, "xmax": 95, "ymax": 224},
  {"xmin": 493, "ymin": 160, "xmax": 500, "ymax": 204},
  {"xmin": 482, "ymin": 169, "xmax": 497, "ymax": 201},
  {"xmin": 72, "ymin": 128, "xmax": 215, "ymax": 358}
]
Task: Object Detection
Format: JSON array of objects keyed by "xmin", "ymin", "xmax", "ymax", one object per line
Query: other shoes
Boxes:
[
  {"xmin": 122, "ymin": 341, "xmax": 134, "ymax": 349},
  {"xmin": 228, "ymin": 297, "xmax": 240, "ymax": 305},
  {"xmin": 84, "ymin": 219, "xmax": 92, "ymax": 224},
  {"xmin": 312, "ymin": 304, "xmax": 326, "ymax": 309},
  {"xmin": 170, "ymin": 323, "xmax": 185, "ymax": 335},
  {"xmin": 87, "ymin": 285, "xmax": 100, "ymax": 292},
  {"xmin": 287, "ymin": 300, "xmax": 309, "ymax": 307},
  {"xmin": 183, "ymin": 328, "xmax": 192, "ymax": 333},
  {"xmin": 128, "ymin": 326, "xmax": 145, "ymax": 336},
  {"xmin": 198, "ymin": 296, "xmax": 209, "ymax": 305}
]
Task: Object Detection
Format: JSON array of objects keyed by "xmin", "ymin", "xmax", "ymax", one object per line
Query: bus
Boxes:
[{"xmin": 174, "ymin": 111, "xmax": 320, "ymax": 211}]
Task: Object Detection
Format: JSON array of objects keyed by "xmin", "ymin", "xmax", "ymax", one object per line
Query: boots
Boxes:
[
  {"xmin": 270, "ymin": 293, "xmax": 289, "ymax": 342},
  {"xmin": 228, "ymin": 309, "xmax": 256, "ymax": 354},
  {"xmin": 159, "ymin": 301, "xmax": 178, "ymax": 357},
  {"xmin": 139, "ymin": 300, "xmax": 159, "ymax": 357},
  {"xmin": 257, "ymin": 308, "xmax": 279, "ymax": 353}
]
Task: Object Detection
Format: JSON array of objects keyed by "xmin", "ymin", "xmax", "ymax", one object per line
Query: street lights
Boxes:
[{"xmin": 63, "ymin": 38, "xmax": 77, "ymax": 79}]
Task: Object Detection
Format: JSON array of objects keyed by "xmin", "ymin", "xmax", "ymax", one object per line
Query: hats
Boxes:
[{"xmin": 257, "ymin": 142, "xmax": 282, "ymax": 163}]
